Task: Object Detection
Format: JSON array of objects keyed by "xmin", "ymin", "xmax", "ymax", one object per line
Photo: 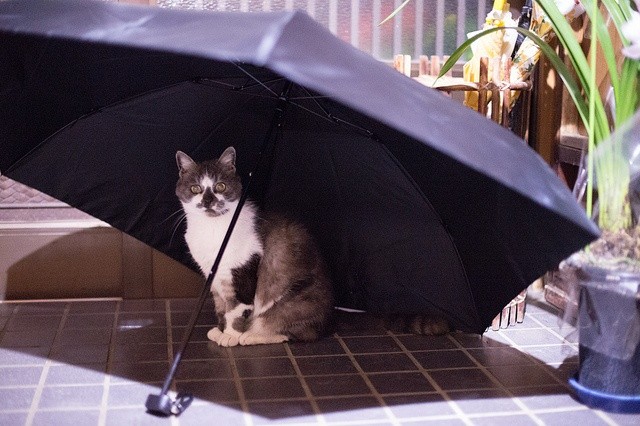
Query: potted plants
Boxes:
[{"xmin": 380, "ymin": 1, "xmax": 640, "ymax": 413}]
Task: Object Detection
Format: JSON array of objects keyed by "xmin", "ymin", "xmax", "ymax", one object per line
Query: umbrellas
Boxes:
[{"xmin": 0, "ymin": 0, "xmax": 602, "ymax": 418}]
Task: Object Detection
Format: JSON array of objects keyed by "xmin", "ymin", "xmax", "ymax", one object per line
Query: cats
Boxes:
[{"xmin": 175, "ymin": 145, "xmax": 450, "ymax": 346}]
